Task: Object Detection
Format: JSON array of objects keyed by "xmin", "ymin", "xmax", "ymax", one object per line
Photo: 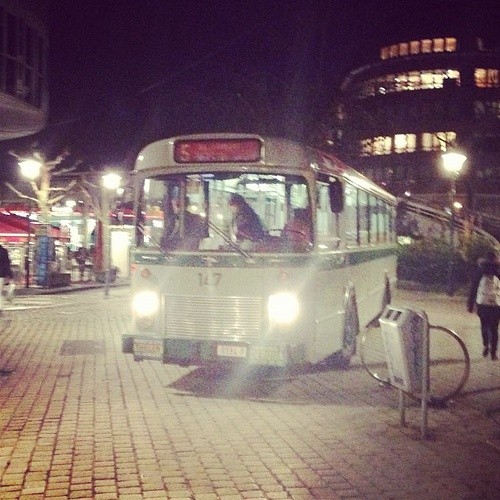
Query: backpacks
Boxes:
[{"xmin": 476, "ymin": 272, "xmax": 500, "ymax": 307}]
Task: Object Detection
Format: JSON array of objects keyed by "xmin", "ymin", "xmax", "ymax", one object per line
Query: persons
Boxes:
[
  {"xmin": 0, "ymin": 243, "xmax": 14, "ymax": 316},
  {"xmin": 164, "ymin": 196, "xmax": 209, "ymax": 252},
  {"xmin": 229, "ymin": 195, "xmax": 271, "ymax": 246},
  {"xmin": 467, "ymin": 252, "xmax": 500, "ymax": 360},
  {"xmin": 280, "ymin": 208, "xmax": 311, "ymax": 248},
  {"xmin": 68, "ymin": 245, "xmax": 96, "ymax": 281}
]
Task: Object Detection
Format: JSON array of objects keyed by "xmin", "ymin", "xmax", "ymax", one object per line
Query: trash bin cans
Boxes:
[{"xmin": 379, "ymin": 305, "xmax": 431, "ymax": 440}]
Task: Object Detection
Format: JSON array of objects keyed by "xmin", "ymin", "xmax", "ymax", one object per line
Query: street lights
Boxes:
[
  {"xmin": 440, "ymin": 152, "xmax": 468, "ymax": 297},
  {"xmin": 18, "ymin": 158, "xmax": 42, "ymax": 288},
  {"xmin": 101, "ymin": 173, "xmax": 121, "ymax": 283}
]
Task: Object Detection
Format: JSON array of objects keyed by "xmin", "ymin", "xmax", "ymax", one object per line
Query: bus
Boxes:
[{"xmin": 117, "ymin": 131, "xmax": 400, "ymax": 379}]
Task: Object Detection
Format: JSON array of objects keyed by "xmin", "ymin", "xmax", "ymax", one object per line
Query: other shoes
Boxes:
[
  {"xmin": 492, "ymin": 354, "xmax": 498, "ymax": 360},
  {"xmin": 483, "ymin": 347, "xmax": 489, "ymax": 356}
]
